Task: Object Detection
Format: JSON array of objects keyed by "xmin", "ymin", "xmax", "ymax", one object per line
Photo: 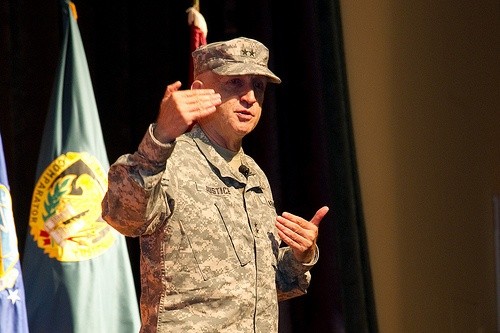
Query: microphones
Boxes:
[{"xmin": 239, "ymin": 165, "xmax": 249, "ymax": 176}]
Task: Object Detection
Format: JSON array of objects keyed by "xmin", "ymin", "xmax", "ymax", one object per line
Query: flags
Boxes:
[{"xmin": 0, "ymin": 0, "xmax": 208, "ymax": 333}]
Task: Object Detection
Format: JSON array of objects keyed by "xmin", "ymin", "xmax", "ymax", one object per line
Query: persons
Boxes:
[{"xmin": 100, "ymin": 37, "xmax": 329, "ymax": 333}]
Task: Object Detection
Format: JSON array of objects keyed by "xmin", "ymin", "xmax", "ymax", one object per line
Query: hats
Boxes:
[{"xmin": 192, "ymin": 37, "xmax": 282, "ymax": 84}]
